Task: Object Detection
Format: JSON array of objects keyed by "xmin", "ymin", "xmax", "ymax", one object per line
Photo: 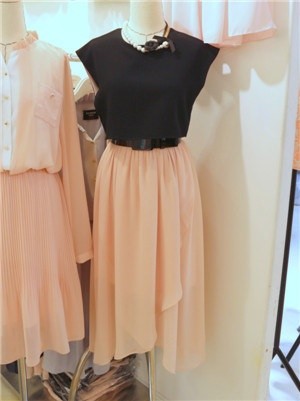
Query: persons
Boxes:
[
  {"xmin": 1, "ymin": 1, "xmax": 93, "ymax": 365},
  {"xmin": 75, "ymin": 1, "xmax": 219, "ymax": 372}
]
